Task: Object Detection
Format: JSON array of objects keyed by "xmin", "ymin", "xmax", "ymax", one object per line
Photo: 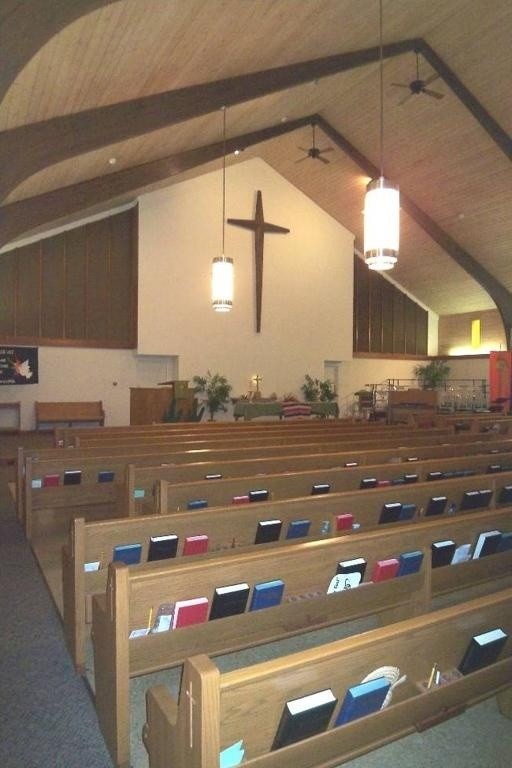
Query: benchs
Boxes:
[
  {"xmin": 8, "ymin": 421, "xmax": 512, "ymax": 768},
  {"xmin": 35, "ymin": 400, "xmax": 106, "ymax": 430}
]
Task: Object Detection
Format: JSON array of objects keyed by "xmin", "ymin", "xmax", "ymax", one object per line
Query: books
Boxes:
[
  {"xmin": 99, "ymin": 472, "xmax": 114, "ymax": 483},
  {"xmin": 44, "ymin": 474, "xmax": 59, "ymax": 486},
  {"xmin": 64, "ymin": 470, "xmax": 82, "ymax": 484}
]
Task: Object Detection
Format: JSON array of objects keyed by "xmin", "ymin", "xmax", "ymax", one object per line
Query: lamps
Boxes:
[
  {"xmin": 211, "ymin": 108, "xmax": 234, "ymax": 313},
  {"xmin": 362, "ymin": 0, "xmax": 400, "ymax": 271}
]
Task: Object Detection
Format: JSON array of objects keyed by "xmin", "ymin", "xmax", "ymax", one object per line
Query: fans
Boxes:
[
  {"xmin": 295, "ymin": 123, "xmax": 333, "ymax": 164},
  {"xmin": 390, "ymin": 50, "xmax": 444, "ymax": 106}
]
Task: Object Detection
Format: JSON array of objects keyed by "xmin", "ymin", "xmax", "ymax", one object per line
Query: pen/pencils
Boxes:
[
  {"xmin": 428, "ymin": 664, "xmax": 441, "ymax": 688},
  {"xmin": 147, "ymin": 606, "xmax": 153, "ymax": 629}
]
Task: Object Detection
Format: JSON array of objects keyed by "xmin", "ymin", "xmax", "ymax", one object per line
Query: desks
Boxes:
[{"xmin": 234, "ymin": 400, "xmax": 338, "ymax": 421}]
